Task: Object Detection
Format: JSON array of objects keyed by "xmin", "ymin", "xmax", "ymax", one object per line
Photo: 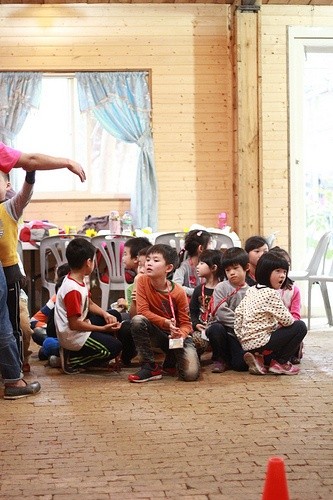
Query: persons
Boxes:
[
  {"xmin": 0, "ymin": 170, "xmax": 35, "ymax": 363},
  {"xmin": 30, "ymin": 230, "xmax": 307, "ymax": 383},
  {"xmin": 0, "ymin": 140, "xmax": 86, "ymax": 400}
]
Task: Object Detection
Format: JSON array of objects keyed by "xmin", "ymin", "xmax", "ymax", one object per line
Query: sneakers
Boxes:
[
  {"xmin": 244, "ymin": 352, "xmax": 268, "ymax": 375},
  {"xmin": 128, "ymin": 367, "xmax": 162, "ymax": 383},
  {"xmin": 268, "ymin": 359, "xmax": 301, "ymax": 375}
]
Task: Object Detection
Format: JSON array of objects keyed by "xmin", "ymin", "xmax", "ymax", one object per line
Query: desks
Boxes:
[{"xmin": 21, "ymin": 241, "xmax": 69, "ymax": 317}]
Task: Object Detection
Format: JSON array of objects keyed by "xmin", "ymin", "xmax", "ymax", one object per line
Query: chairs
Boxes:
[
  {"xmin": 41, "ymin": 226, "xmax": 274, "ymax": 312},
  {"xmin": 287, "ymin": 233, "xmax": 333, "ymax": 330}
]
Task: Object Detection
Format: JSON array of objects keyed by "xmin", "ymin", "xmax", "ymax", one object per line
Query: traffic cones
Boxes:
[{"xmin": 260, "ymin": 456, "xmax": 290, "ymax": 500}]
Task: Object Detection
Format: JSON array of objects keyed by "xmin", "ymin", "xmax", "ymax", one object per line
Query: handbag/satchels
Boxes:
[
  {"xmin": 82, "ymin": 214, "xmax": 135, "ymax": 234},
  {"xmin": 19, "ymin": 221, "xmax": 65, "ymax": 250}
]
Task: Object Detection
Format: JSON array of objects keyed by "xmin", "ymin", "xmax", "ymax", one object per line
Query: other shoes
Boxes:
[
  {"xmin": 3, "ymin": 378, "xmax": 41, "ymax": 400},
  {"xmin": 211, "ymin": 362, "xmax": 225, "ymax": 373},
  {"xmin": 50, "ymin": 356, "xmax": 61, "ymax": 368},
  {"xmin": 22, "ymin": 363, "xmax": 31, "ymax": 373},
  {"xmin": 60, "ymin": 348, "xmax": 80, "ymax": 374}
]
